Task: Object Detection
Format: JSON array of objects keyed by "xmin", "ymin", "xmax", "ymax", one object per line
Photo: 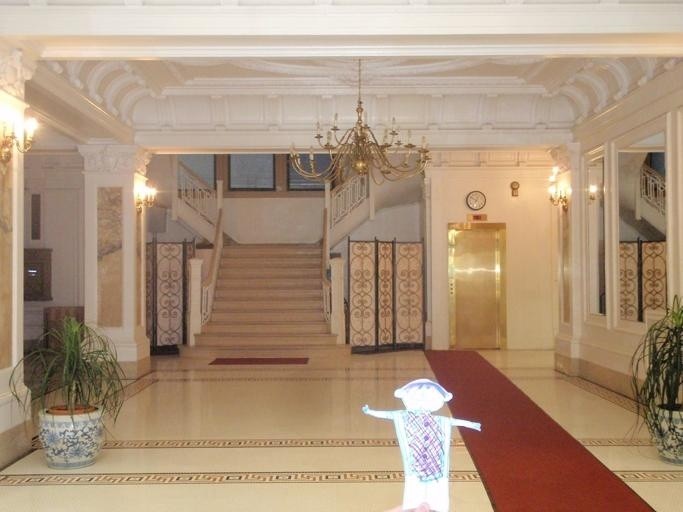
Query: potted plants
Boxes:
[
  {"xmin": 9, "ymin": 315, "xmax": 126, "ymax": 469},
  {"xmin": 629, "ymin": 295, "xmax": 683, "ymax": 463}
]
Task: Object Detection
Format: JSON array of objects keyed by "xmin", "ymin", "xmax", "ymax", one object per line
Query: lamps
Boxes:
[
  {"xmin": 290, "ymin": 60, "xmax": 429, "ymax": 183},
  {"xmin": 134, "ymin": 183, "xmax": 157, "ymax": 214},
  {"xmin": 549, "ymin": 167, "xmax": 572, "ymax": 212},
  {"xmin": 1, "ymin": 107, "xmax": 37, "ymax": 174},
  {"xmin": 590, "ymin": 183, "xmax": 597, "ymax": 201}
]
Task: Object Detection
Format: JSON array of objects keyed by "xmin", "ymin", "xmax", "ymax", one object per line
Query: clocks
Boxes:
[{"xmin": 466, "ymin": 191, "xmax": 486, "ymax": 210}]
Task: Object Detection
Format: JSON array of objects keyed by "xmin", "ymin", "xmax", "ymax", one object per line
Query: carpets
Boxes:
[{"xmin": 209, "ymin": 357, "xmax": 309, "ymax": 364}]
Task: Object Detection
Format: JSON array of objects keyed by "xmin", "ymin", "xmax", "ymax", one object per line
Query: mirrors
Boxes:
[
  {"xmin": 617, "ymin": 130, "xmax": 667, "ymax": 322},
  {"xmin": 585, "ymin": 158, "xmax": 606, "ymax": 315}
]
